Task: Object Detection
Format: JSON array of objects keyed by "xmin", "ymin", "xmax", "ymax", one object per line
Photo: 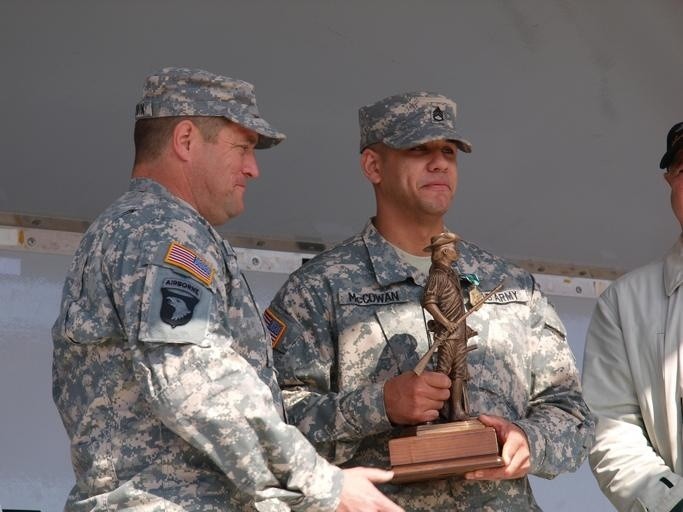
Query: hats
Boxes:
[
  {"xmin": 659, "ymin": 121, "xmax": 683, "ymax": 169},
  {"xmin": 359, "ymin": 91, "xmax": 473, "ymax": 153},
  {"xmin": 134, "ymin": 66, "xmax": 286, "ymax": 150}
]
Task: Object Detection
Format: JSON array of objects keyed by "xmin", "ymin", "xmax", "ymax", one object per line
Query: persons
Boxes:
[
  {"xmin": 48, "ymin": 63, "xmax": 410, "ymax": 512},
  {"xmin": 421, "ymin": 233, "xmax": 483, "ymax": 423},
  {"xmin": 579, "ymin": 120, "xmax": 683, "ymax": 512},
  {"xmin": 262, "ymin": 93, "xmax": 597, "ymax": 512}
]
